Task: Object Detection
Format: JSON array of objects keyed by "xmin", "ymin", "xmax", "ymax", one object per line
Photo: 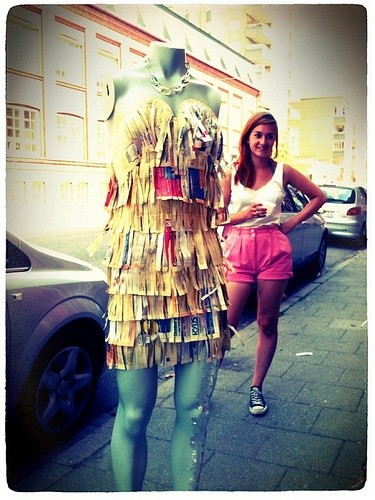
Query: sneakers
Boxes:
[{"xmin": 249, "ymin": 385, "xmax": 267, "ymax": 415}]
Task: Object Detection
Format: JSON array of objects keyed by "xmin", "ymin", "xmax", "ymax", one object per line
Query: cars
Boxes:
[
  {"xmin": 7, "ymin": 228, "xmax": 113, "ymax": 460},
  {"xmin": 308, "ymin": 182, "xmax": 367, "ymax": 251},
  {"xmin": 273, "ymin": 183, "xmax": 329, "ymax": 288}
]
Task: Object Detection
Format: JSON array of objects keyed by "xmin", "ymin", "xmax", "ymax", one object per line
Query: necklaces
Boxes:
[{"xmin": 142, "ymin": 55, "xmax": 192, "ymax": 96}]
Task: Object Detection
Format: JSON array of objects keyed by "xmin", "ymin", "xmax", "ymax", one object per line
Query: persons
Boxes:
[
  {"xmin": 93, "ymin": 38, "xmax": 225, "ymax": 491},
  {"xmin": 215, "ymin": 109, "xmax": 328, "ymax": 418}
]
